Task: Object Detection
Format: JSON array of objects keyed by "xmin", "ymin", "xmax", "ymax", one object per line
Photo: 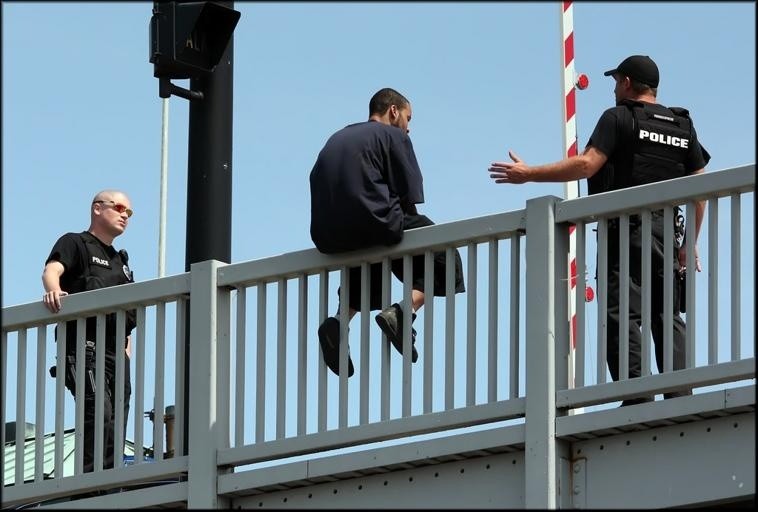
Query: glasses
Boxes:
[{"xmin": 92, "ymin": 200, "xmax": 133, "ymax": 217}]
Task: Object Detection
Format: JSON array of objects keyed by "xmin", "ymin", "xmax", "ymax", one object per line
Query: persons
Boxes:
[
  {"xmin": 308, "ymin": 86, "xmax": 466, "ymax": 378},
  {"xmin": 42, "ymin": 188, "xmax": 137, "ymax": 474},
  {"xmin": 487, "ymin": 55, "xmax": 711, "ymax": 408}
]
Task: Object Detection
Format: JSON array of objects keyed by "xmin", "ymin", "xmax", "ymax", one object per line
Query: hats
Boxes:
[{"xmin": 602, "ymin": 54, "xmax": 660, "ymax": 91}]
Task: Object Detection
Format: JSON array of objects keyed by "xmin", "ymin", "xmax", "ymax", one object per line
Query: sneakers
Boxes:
[
  {"xmin": 374, "ymin": 301, "xmax": 420, "ymax": 364},
  {"xmin": 317, "ymin": 318, "xmax": 355, "ymax": 379}
]
